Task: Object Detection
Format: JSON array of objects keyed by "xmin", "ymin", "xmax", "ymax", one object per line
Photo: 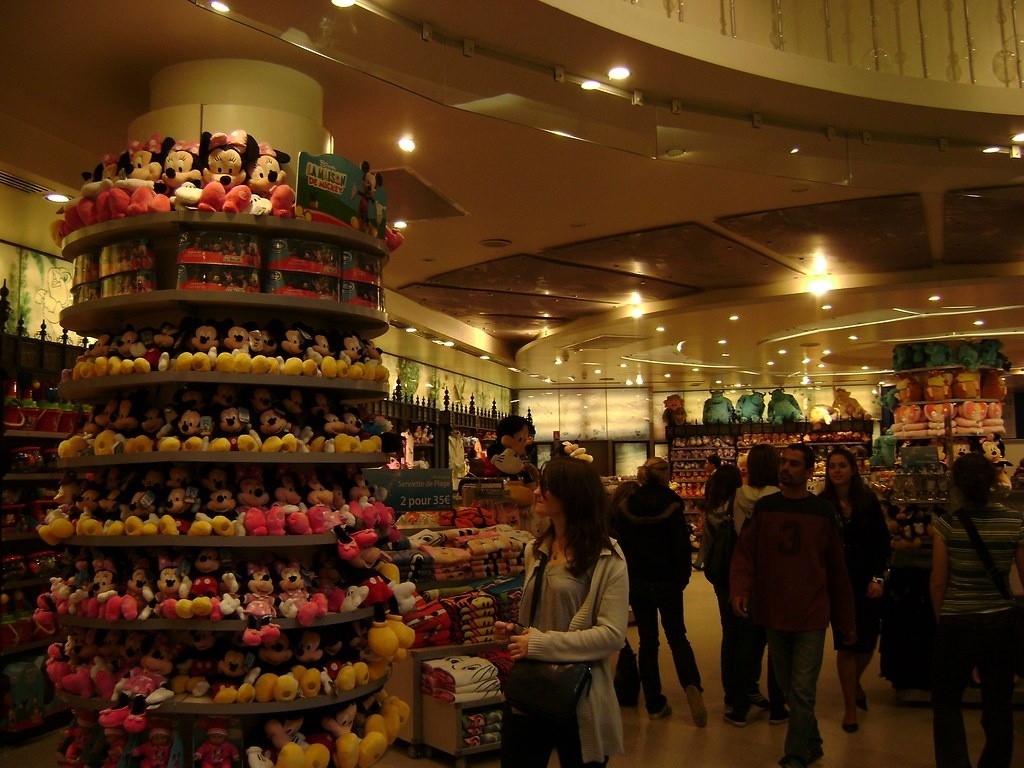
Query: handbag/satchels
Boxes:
[
  {"xmin": 705, "ymin": 519, "xmax": 737, "ymax": 585},
  {"xmin": 614, "ymin": 639, "xmax": 641, "ymax": 707},
  {"xmin": 1008, "ymin": 605, "xmax": 1023, "ymax": 675},
  {"xmin": 502, "ymin": 657, "xmax": 591, "ymax": 726}
]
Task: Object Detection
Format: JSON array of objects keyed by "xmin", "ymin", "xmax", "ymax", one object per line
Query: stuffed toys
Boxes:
[
  {"xmin": 871, "ymin": 338, "xmax": 1012, "ymax": 548},
  {"xmin": 39, "ymin": 463, "xmax": 411, "ymax": 556},
  {"xmin": 51, "ymin": 129, "xmax": 405, "ymax": 251},
  {"xmin": 45, "ymin": 627, "xmax": 410, "ymax": 768},
  {"xmin": 490, "ymin": 415, "xmax": 533, "ymax": 474},
  {"xmin": 413, "ymin": 425, "xmax": 434, "ymax": 443},
  {"xmin": 670, "ymin": 431, "xmax": 869, "ymax": 547},
  {"xmin": 33, "ymin": 547, "xmax": 415, "ymax": 657},
  {"xmin": 561, "ymin": 441, "xmax": 593, "ymax": 462},
  {"xmin": 61, "ymin": 317, "xmax": 389, "ymax": 382},
  {"xmin": 58, "ymin": 388, "xmax": 402, "ymax": 458}
]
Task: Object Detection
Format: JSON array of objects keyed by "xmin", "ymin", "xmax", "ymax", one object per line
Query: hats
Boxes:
[{"xmin": 953, "ymin": 453, "xmax": 994, "ymax": 495}]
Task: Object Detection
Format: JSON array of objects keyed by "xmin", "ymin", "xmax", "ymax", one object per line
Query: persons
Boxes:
[
  {"xmin": 493, "ymin": 457, "xmax": 629, "ymax": 768},
  {"xmin": 691, "ymin": 444, "xmax": 791, "ymax": 726},
  {"xmin": 931, "ymin": 454, "xmax": 1024, "ymax": 768},
  {"xmin": 817, "ymin": 449, "xmax": 890, "ymax": 732},
  {"xmin": 609, "ymin": 456, "xmax": 708, "ymax": 727},
  {"xmin": 730, "ymin": 443, "xmax": 856, "ymax": 768}
]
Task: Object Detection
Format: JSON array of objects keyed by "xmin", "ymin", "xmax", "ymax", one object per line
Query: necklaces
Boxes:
[{"xmin": 551, "ymin": 545, "xmax": 560, "ymax": 560}]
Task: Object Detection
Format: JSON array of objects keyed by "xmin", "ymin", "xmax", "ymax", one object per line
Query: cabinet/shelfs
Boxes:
[
  {"xmin": 665, "ymin": 421, "xmax": 874, "ymax": 553},
  {"xmin": 894, "ymin": 366, "xmax": 1005, "ymax": 439},
  {"xmin": 357, "ymin": 379, "xmax": 532, "ymax": 468},
  {"xmin": 383, "ymin": 525, "xmax": 506, "ymax": 768},
  {"xmin": 888, "ymin": 472, "xmax": 1024, "ymax": 703},
  {"xmin": 54, "ymin": 211, "xmax": 387, "ymax": 714},
  {"xmin": 0, "ymin": 428, "xmax": 73, "ymax": 747}
]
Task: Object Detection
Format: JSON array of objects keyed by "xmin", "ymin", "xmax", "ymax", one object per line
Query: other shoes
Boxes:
[
  {"xmin": 751, "ymin": 694, "xmax": 770, "ymax": 710},
  {"xmin": 858, "ymin": 696, "xmax": 869, "ymax": 711},
  {"xmin": 769, "ymin": 709, "xmax": 790, "ymax": 723},
  {"xmin": 686, "ymin": 685, "xmax": 707, "ymax": 727},
  {"xmin": 843, "ymin": 723, "xmax": 859, "ymax": 734},
  {"xmin": 778, "ymin": 746, "xmax": 822, "ymax": 768},
  {"xmin": 648, "ymin": 705, "xmax": 672, "ymax": 719},
  {"xmin": 724, "ymin": 711, "xmax": 748, "ymax": 726}
]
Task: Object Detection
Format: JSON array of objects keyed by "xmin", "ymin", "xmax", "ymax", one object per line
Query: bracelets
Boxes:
[{"xmin": 872, "ymin": 578, "xmax": 884, "ymax": 585}]
